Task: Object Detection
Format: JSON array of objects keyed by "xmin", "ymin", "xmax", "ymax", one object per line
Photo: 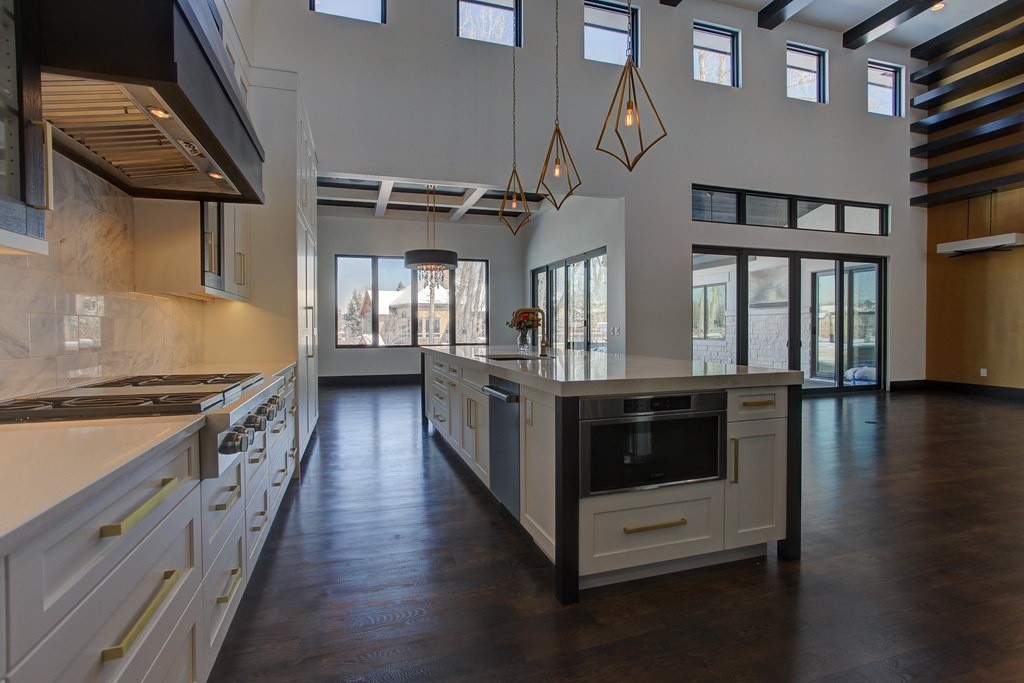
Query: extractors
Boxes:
[{"xmin": 40, "ymin": 0, "xmax": 265, "ymax": 205}]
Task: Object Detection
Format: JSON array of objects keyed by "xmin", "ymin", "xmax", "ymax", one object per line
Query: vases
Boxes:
[{"xmin": 517, "ymin": 329, "xmax": 530, "ymax": 350}]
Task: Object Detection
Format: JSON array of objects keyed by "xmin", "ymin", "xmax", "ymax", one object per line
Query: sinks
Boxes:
[{"xmin": 474, "ymin": 354, "xmax": 551, "ymax": 361}]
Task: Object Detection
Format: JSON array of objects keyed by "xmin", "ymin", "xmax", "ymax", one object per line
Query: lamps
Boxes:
[
  {"xmin": 498, "ymin": 0, "xmax": 531, "ymax": 236},
  {"xmin": 536, "ymin": 0, "xmax": 581, "ymax": 212},
  {"xmin": 404, "ymin": 185, "xmax": 457, "ymax": 301},
  {"xmin": 595, "ymin": 0, "xmax": 668, "ymax": 173}
]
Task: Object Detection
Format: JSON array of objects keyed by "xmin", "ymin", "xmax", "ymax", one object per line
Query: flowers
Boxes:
[{"xmin": 506, "ymin": 311, "xmax": 542, "ymax": 347}]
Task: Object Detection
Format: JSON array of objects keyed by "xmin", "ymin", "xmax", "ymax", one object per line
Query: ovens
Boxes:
[{"xmin": 579, "ymin": 388, "xmax": 729, "ymax": 499}]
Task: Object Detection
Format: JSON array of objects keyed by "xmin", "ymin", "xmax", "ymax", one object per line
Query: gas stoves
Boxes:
[{"xmin": 0, "ymin": 373, "xmax": 286, "ymax": 479}]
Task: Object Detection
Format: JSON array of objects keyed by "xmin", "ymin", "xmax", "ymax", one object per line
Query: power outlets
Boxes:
[{"xmin": 980, "ymin": 369, "xmax": 987, "ymax": 376}]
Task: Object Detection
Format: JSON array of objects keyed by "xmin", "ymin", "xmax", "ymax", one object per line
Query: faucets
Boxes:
[{"xmin": 514, "ymin": 308, "xmax": 547, "ymax": 356}]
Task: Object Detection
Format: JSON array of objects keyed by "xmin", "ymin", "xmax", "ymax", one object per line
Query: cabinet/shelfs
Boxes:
[
  {"xmin": 0, "ymin": 0, "xmax": 54, "ymax": 256},
  {"xmin": 419, "ymin": 346, "xmax": 801, "ymax": 605},
  {"xmin": 909, "ymin": 0, "xmax": 1024, "ymax": 253},
  {"xmin": 133, "ymin": 198, "xmax": 250, "ymax": 304},
  {"xmin": 205, "ymin": 64, "xmax": 318, "ymax": 461},
  {"xmin": 0, "ymin": 362, "xmax": 315, "ymax": 683}
]
